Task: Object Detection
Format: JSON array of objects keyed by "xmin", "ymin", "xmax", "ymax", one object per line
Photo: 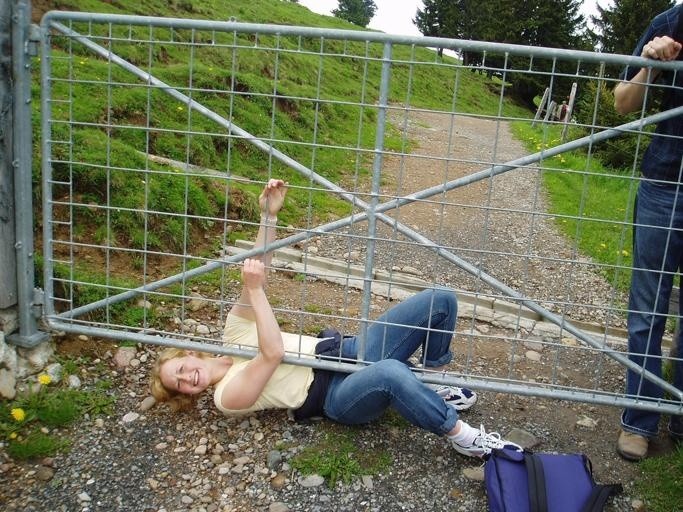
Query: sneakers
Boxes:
[
  {"xmin": 617, "ymin": 430, "xmax": 650, "ymax": 462},
  {"xmin": 436, "ymin": 386, "xmax": 478, "ymax": 411},
  {"xmin": 452, "ymin": 424, "xmax": 524, "ymax": 461}
]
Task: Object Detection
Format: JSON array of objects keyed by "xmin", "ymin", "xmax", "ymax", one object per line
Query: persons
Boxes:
[
  {"xmin": 149, "ymin": 179, "xmax": 525, "ymax": 460},
  {"xmin": 614, "ymin": 2, "xmax": 683, "ymax": 461},
  {"xmin": 556, "ymin": 101, "xmax": 569, "ymax": 121}
]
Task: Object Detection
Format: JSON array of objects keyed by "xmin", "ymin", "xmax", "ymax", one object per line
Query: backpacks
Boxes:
[{"xmin": 484, "ymin": 445, "xmax": 623, "ymax": 512}]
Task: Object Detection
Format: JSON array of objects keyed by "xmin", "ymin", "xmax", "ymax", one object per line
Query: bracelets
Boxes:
[{"xmin": 260, "ymin": 214, "xmax": 278, "ymax": 222}]
